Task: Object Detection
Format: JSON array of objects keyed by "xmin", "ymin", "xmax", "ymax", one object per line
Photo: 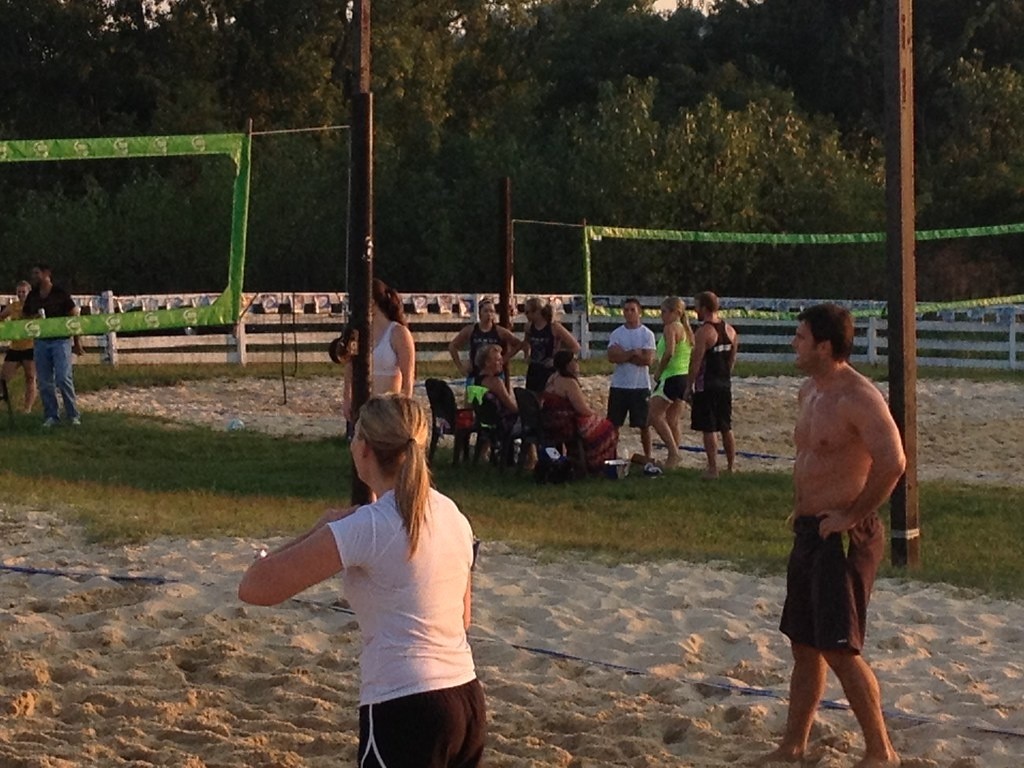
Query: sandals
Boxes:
[{"xmin": 644, "ymin": 463, "xmax": 663, "ymax": 475}]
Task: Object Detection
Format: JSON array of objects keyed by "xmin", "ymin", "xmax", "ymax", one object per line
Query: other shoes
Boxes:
[
  {"xmin": 43, "ymin": 422, "xmax": 51, "ymax": 427},
  {"xmin": 72, "ymin": 417, "xmax": 81, "ymax": 425}
]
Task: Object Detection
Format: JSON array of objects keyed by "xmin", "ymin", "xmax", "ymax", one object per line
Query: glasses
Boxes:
[{"xmin": 524, "ymin": 310, "xmax": 535, "ymax": 314}]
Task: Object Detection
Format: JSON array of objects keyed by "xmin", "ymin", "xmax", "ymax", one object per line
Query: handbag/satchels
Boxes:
[
  {"xmin": 539, "ymin": 443, "xmax": 574, "ymax": 483},
  {"xmin": 649, "ymin": 380, "xmax": 673, "ymax": 425}
]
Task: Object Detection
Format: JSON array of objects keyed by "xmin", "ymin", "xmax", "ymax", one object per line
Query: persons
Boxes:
[
  {"xmin": 236, "ymin": 391, "xmax": 487, "ymax": 767},
  {"xmin": 745, "ymin": 303, "xmax": 908, "ymax": 766},
  {"xmin": 0, "ymin": 265, "xmax": 87, "ymax": 426},
  {"xmin": 330, "ymin": 279, "xmax": 739, "ymax": 486}
]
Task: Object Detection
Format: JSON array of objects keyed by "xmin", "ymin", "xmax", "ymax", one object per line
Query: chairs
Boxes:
[
  {"xmin": 425, "ymin": 378, "xmax": 479, "ymax": 460},
  {"xmin": 513, "ymin": 387, "xmax": 588, "ymax": 483},
  {"xmin": 467, "ymin": 386, "xmax": 525, "ymax": 468}
]
